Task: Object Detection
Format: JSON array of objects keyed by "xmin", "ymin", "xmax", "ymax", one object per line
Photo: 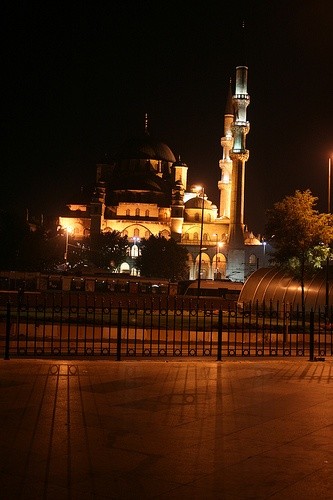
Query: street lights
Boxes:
[
  {"xmin": 188, "ymin": 184, "xmax": 205, "ymax": 298},
  {"xmin": 64, "ymin": 226, "xmax": 73, "ymax": 264}
]
